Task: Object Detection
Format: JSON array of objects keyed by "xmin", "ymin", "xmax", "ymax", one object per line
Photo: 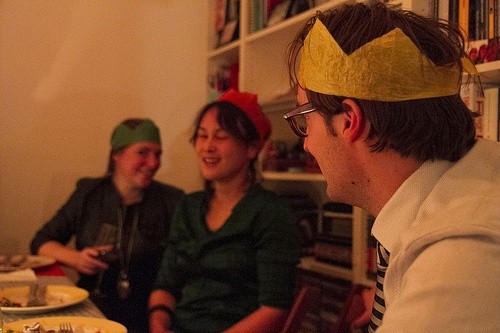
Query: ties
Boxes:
[{"xmin": 367, "ymin": 240, "xmax": 390, "ymax": 333}]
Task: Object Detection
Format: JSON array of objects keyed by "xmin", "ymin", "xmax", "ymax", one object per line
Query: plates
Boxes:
[
  {"xmin": 0, "ymin": 285, "xmax": 90, "ymax": 314},
  {"xmin": 0, "ymin": 316, "xmax": 128, "ymax": 333},
  {"xmin": 0, "ymin": 254, "xmax": 56, "ymax": 271}
]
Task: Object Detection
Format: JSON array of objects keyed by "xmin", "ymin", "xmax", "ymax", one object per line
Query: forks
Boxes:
[{"xmin": 59, "ymin": 322, "xmax": 73, "ymax": 333}]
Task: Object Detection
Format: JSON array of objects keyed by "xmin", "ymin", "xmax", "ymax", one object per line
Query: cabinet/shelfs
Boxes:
[{"xmin": 207, "ymin": 0, "xmax": 500, "ymax": 285}]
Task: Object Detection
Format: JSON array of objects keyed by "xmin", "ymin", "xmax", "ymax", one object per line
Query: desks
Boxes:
[{"xmin": 0, "ymin": 261, "xmax": 126, "ymax": 333}]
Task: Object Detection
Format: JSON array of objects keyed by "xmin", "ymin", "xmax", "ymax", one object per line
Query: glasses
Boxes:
[{"xmin": 281, "ymin": 101, "xmax": 316, "ymax": 138}]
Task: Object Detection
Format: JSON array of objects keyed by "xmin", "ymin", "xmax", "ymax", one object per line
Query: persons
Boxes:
[
  {"xmin": 283, "ymin": 0, "xmax": 500, "ymax": 333},
  {"xmin": 29, "ymin": 116, "xmax": 187, "ymax": 333},
  {"xmin": 145, "ymin": 88, "xmax": 298, "ymax": 333}
]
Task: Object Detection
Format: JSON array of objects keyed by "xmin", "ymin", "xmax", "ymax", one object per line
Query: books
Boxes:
[
  {"xmin": 458, "ymin": 82, "xmax": 500, "ymax": 142},
  {"xmin": 214, "ymin": 0, "xmax": 314, "ymax": 48},
  {"xmin": 277, "ymin": 190, "xmax": 378, "ymax": 333},
  {"xmin": 427, "ymin": 1, "xmax": 500, "ymax": 66}
]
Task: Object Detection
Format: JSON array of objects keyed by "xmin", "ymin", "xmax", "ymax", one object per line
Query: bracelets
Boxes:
[{"xmin": 148, "ymin": 305, "xmax": 175, "ymax": 316}]
